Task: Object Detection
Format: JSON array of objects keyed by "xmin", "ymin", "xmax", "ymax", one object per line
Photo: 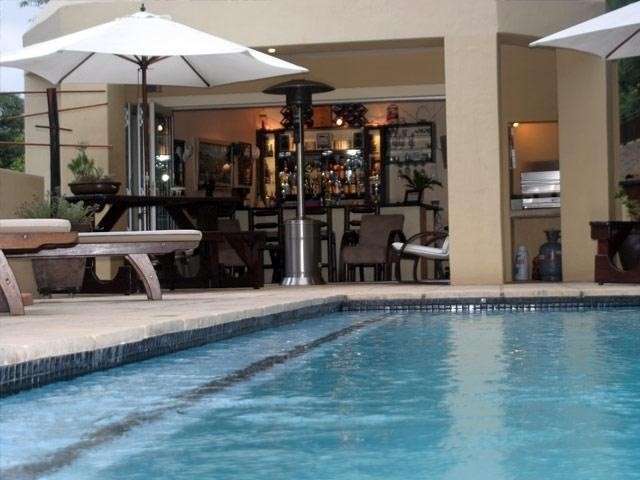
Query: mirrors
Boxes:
[
  {"xmin": 304, "ymin": 129, "xmax": 381, "ymax": 154},
  {"xmin": 509, "ymin": 120, "xmax": 560, "ymax": 199}
]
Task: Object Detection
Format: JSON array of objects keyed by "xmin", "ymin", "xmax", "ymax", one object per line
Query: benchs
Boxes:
[
  {"xmin": 0, "ymin": 218, "xmax": 78, "ymax": 315},
  {"xmin": 122, "ymin": 232, "xmax": 265, "ymax": 294},
  {"xmin": 6, "ymin": 229, "xmax": 202, "ymax": 300}
]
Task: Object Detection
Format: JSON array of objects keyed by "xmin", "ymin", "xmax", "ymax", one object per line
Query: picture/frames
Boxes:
[
  {"xmin": 195, "ymin": 136, "xmax": 233, "ymax": 187},
  {"xmin": 234, "ymin": 140, "xmax": 256, "ymax": 187}
]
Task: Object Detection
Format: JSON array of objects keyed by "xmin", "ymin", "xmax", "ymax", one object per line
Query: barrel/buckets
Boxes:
[{"xmin": 538, "ymin": 230, "xmax": 562, "ymax": 281}]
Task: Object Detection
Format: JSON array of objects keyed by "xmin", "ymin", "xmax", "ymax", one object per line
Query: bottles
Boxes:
[
  {"xmin": 514, "ymin": 244, "xmax": 530, "ymax": 280},
  {"xmin": 204, "ymin": 175, "xmax": 215, "ymax": 196},
  {"xmin": 280, "ymin": 157, "xmax": 379, "ymax": 202}
]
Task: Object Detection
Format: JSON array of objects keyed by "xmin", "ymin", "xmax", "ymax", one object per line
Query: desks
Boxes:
[{"xmin": 65, "ymin": 187, "xmax": 266, "ymax": 295}]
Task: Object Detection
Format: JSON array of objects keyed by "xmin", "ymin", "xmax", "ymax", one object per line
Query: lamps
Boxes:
[
  {"xmin": 331, "ymin": 102, "xmax": 369, "ymax": 128},
  {"xmin": 156, "ymin": 119, "xmax": 171, "ymax": 181},
  {"xmin": 262, "ymin": 79, "xmax": 335, "ymax": 286}
]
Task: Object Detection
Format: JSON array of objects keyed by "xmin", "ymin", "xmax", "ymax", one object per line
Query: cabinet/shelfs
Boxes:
[
  {"xmin": 256, "ymin": 125, "xmax": 385, "ymax": 206},
  {"xmin": 385, "ymin": 121, "xmax": 436, "ymax": 167}
]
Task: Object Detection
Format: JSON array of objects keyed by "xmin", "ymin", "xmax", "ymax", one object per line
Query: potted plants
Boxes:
[
  {"xmin": 67, "ymin": 140, "xmax": 121, "ymax": 195},
  {"xmin": 14, "ymin": 186, "xmax": 99, "ymax": 295},
  {"xmin": 398, "ymin": 168, "xmax": 443, "ymax": 202}
]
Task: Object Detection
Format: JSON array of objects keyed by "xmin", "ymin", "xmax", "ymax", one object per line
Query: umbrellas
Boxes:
[
  {"xmin": 0, "ymin": 3, "xmax": 309, "ymax": 228},
  {"xmin": 529, "ymin": 1, "xmax": 640, "ymax": 63}
]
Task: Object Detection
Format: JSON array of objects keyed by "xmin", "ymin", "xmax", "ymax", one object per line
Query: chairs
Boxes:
[
  {"xmin": 296, "ymin": 206, "xmax": 337, "ymax": 282},
  {"xmin": 339, "ymin": 215, "xmax": 406, "ymax": 282},
  {"xmin": 248, "ymin": 207, "xmax": 285, "ymax": 284},
  {"xmin": 392, "ymin": 232, "xmax": 449, "ymax": 284},
  {"xmin": 345, "ymin": 206, "xmax": 380, "ymax": 282}
]
